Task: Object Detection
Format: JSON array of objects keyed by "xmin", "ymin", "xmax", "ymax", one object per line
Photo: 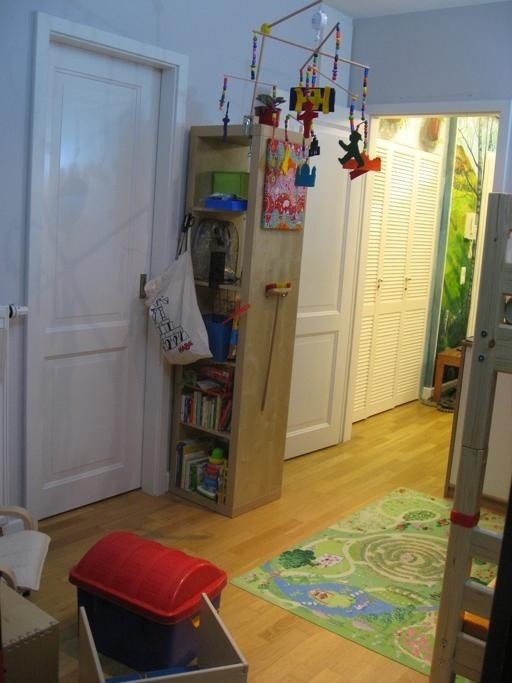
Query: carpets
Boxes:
[{"xmin": 227, "ymin": 486, "xmax": 504, "ymax": 682}]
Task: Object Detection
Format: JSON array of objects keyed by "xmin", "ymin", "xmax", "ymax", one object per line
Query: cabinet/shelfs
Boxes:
[
  {"xmin": 350, "ymin": 137, "xmax": 444, "ymax": 423},
  {"xmin": 169, "ymin": 121, "xmax": 312, "ymax": 519}
]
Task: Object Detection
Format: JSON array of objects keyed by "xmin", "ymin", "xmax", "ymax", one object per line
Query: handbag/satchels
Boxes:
[{"xmin": 144, "ymin": 225, "xmax": 215, "ymax": 365}]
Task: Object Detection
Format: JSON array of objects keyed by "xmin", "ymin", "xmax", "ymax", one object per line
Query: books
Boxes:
[{"xmin": 174, "ymin": 364, "xmax": 235, "ymax": 505}]
